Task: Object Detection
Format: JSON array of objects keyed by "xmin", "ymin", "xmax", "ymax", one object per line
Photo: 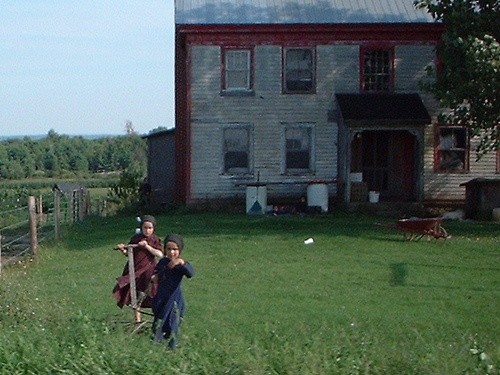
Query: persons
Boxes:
[
  {"xmin": 148, "ymin": 231, "xmax": 195, "ymax": 353},
  {"xmin": 112, "ymin": 214, "xmax": 168, "ymax": 324}
]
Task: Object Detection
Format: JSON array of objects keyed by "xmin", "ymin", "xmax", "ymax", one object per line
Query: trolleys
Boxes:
[{"xmin": 374, "ymin": 215, "xmax": 449, "ymax": 243}]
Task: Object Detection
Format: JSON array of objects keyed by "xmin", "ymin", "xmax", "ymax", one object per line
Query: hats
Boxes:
[
  {"xmin": 163, "ymin": 233, "xmax": 184, "ymax": 254},
  {"xmin": 140, "ymin": 215, "xmax": 157, "ymax": 232}
]
